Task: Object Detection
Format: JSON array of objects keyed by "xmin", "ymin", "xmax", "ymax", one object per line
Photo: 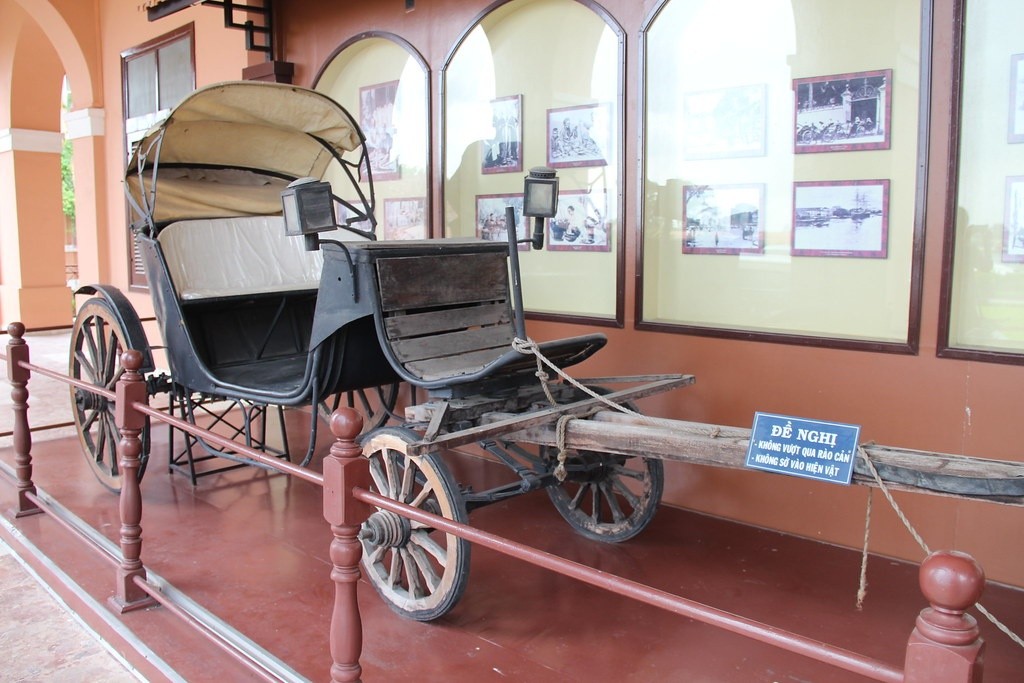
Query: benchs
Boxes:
[{"xmin": 156, "ymin": 215, "xmax": 331, "ymax": 308}]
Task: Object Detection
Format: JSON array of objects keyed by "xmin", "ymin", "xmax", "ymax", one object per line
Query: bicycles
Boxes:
[{"xmin": 794, "ymin": 116, "xmax": 861, "ymax": 142}]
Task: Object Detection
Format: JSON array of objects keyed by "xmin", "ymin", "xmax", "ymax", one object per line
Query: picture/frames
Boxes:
[
  {"xmin": 546, "ymin": 101, "xmax": 613, "ymax": 169},
  {"xmin": 545, "ymin": 189, "xmax": 611, "ymax": 253},
  {"xmin": 790, "ymin": 179, "xmax": 890, "ymax": 259},
  {"xmin": 480, "ymin": 94, "xmax": 524, "ymax": 175},
  {"xmin": 1006, "ymin": 53, "xmax": 1024, "ymax": 144},
  {"xmin": 682, "ymin": 82, "xmax": 767, "ymax": 161},
  {"xmin": 336, "ymin": 199, "xmax": 376, "ymax": 231},
  {"xmin": 792, "ymin": 67, "xmax": 893, "ymax": 154},
  {"xmin": 474, "ymin": 193, "xmax": 531, "ymax": 251},
  {"xmin": 358, "ymin": 80, "xmax": 402, "ymax": 182},
  {"xmin": 1001, "ymin": 175, "xmax": 1024, "ymax": 264},
  {"xmin": 682, "ymin": 182, "xmax": 765, "ymax": 255},
  {"xmin": 383, "ymin": 197, "xmax": 427, "ymax": 240}
]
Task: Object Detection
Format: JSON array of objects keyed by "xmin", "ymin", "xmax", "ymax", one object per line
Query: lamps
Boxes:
[
  {"xmin": 516, "ymin": 166, "xmax": 560, "ymax": 250},
  {"xmin": 281, "ymin": 177, "xmax": 359, "ymax": 303}
]
